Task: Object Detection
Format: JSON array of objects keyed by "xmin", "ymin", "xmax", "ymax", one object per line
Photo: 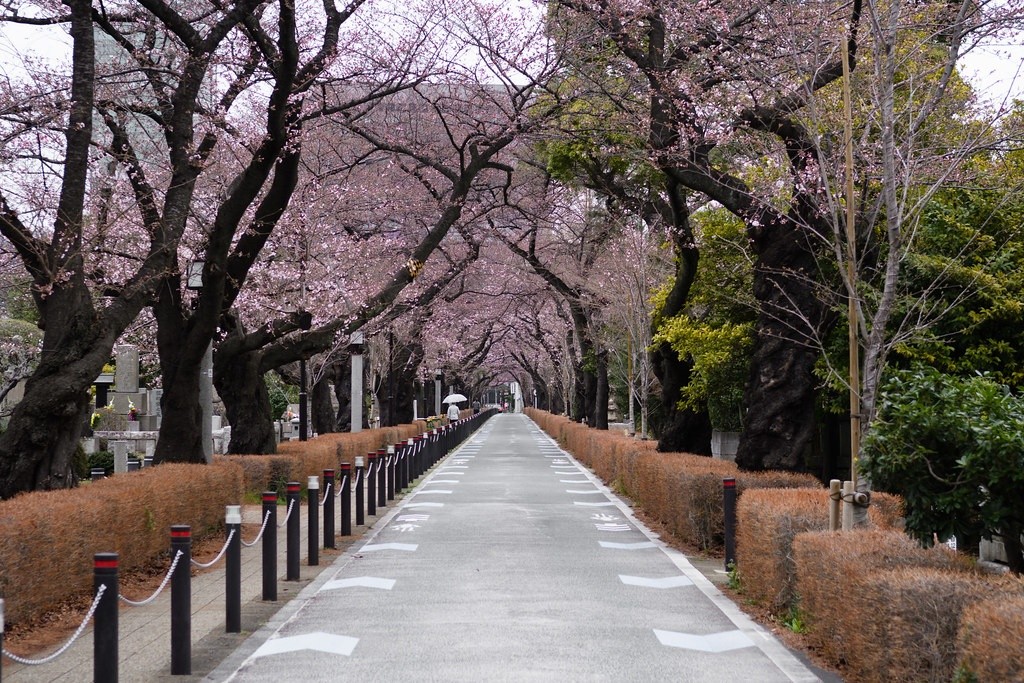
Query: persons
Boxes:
[
  {"xmin": 445, "ymin": 402, "xmax": 460, "ymax": 423},
  {"xmin": 472, "ymin": 398, "xmax": 481, "ymax": 415},
  {"xmin": 498, "ymin": 400, "xmax": 511, "ymax": 413}
]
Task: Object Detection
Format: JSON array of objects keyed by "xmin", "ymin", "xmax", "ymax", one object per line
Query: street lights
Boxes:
[
  {"xmin": 434, "ymin": 369, "xmax": 441, "ymax": 416},
  {"xmin": 350, "ymin": 331, "xmax": 364, "ymax": 436}
]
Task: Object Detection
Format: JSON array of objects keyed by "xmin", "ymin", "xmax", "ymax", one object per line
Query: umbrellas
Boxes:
[{"xmin": 442, "ymin": 393, "xmax": 468, "ymax": 404}]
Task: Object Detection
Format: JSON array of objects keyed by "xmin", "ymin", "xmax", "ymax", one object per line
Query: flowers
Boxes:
[{"xmin": 0, "ymin": 0, "xmax": 1024, "ymax": 405}]
